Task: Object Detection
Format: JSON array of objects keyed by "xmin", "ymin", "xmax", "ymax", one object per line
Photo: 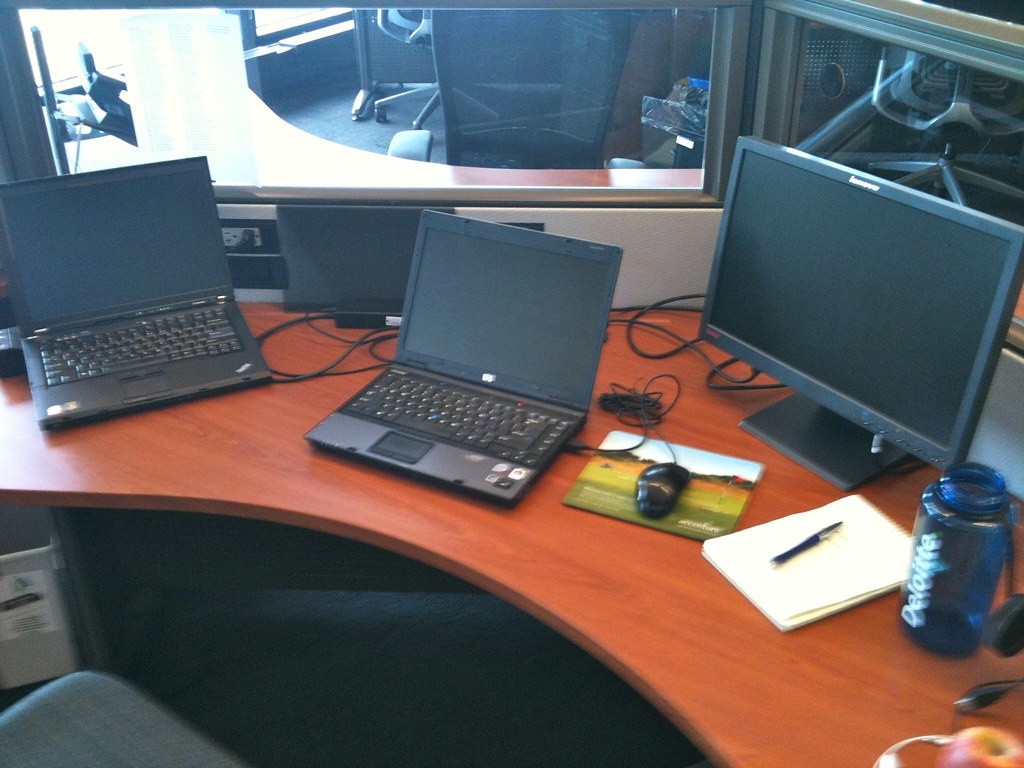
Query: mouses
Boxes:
[
  {"xmin": 982, "ymin": 592, "xmax": 1024, "ymax": 658},
  {"xmin": 635, "ymin": 462, "xmax": 690, "ymax": 519}
]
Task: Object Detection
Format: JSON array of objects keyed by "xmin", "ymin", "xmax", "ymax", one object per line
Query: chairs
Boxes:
[
  {"xmin": 388, "ymin": 9, "xmax": 648, "ymax": 170},
  {"xmin": 374, "ymin": 7, "xmax": 441, "ymax": 129},
  {"xmin": 866, "ymin": 44, "xmax": 1024, "ymax": 216}
]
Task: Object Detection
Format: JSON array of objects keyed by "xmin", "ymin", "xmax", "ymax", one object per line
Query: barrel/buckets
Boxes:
[{"xmin": 0, "ymin": 504, "xmax": 75, "ymax": 689}]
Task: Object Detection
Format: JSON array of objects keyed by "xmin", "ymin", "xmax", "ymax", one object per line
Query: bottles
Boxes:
[{"xmin": 901, "ymin": 460, "xmax": 1013, "ymax": 657}]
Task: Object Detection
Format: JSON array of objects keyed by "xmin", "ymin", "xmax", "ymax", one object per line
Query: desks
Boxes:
[
  {"xmin": 0, "ymin": 301, "xmax": 1024, "ymax": 768},
  {"xmin": 0, "ymin": 84, "xmax": 705, "ymax": 190}
]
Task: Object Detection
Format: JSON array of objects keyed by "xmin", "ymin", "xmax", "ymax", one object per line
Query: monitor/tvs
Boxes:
[{"xmin": 698, "ymin": 136, "xmax": 1024, "ymax": 493}]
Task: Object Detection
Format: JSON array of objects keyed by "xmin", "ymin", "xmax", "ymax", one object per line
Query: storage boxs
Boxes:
[{"xmin": 0, "ymin": 503, "xmax": 87, "ymax": 699}]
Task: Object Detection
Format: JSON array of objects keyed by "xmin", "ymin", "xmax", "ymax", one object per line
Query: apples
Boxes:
[{"xmin": 935, "ymin": 726, "xmax": 1024, "ymax": 768}]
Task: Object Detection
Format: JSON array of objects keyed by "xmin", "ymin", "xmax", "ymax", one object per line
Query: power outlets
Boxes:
[{"xmin": 221, "ymin": 228, "xmax": 261, "ymax": 248}]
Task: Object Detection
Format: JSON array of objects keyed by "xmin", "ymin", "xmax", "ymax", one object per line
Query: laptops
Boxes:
[
  {"xmin": 306, "ymin": 207, "xmax": 625, "ymax": 508},
  {"xmin": 0, "ymin": 156, "xmax": 274, "ymax": 429}
]
